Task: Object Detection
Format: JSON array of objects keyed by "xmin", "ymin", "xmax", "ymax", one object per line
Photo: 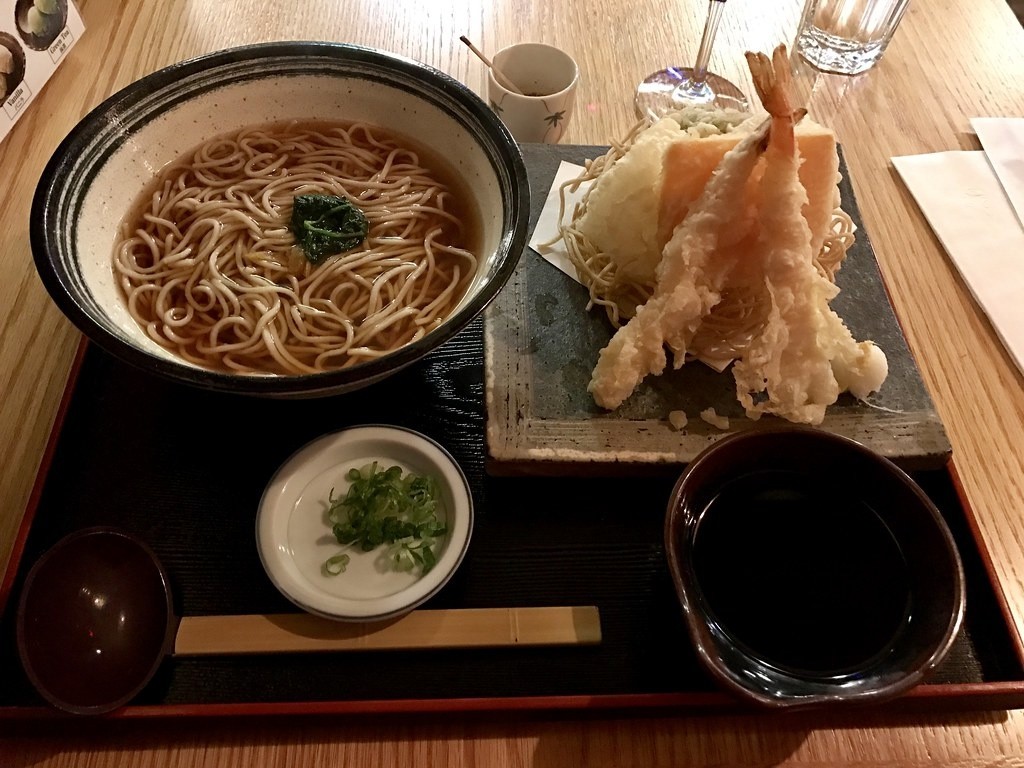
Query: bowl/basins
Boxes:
[
  {"xmin": 29, "ymin": 39, "xmax": 534, "ymax": 404},
  {"xmin": 662, "ymin": 424, "xmax": 966, "ymax": 710},
  {"xmin": 17, "ymin": 529, "xmax": 177, "ymax": 720}
]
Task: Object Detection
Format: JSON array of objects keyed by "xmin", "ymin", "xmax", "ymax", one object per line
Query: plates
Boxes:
[
  {"xmin": 482, "ymin": 139, "xmax": 954, "ymax": 460},
  {"xmin": 252, "ymin": 423, "xmax": 475, "ymax": 621}
]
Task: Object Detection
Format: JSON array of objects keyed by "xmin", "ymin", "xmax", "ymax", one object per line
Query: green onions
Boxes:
[{"xmin": 325, "ymin": 462, "xmax": 446, "ymax": 576}]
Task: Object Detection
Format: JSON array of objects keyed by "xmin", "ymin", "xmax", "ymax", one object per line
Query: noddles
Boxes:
[{"xmin": 113, "ymin": 124, "xmax": 477, "ymax": 375}]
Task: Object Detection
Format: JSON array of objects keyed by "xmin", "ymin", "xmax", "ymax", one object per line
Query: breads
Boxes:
[{"xmin": 580, "ymin": 111, "xmax": 837, "ymax": 281}]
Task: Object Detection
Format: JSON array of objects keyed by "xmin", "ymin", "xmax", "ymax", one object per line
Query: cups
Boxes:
[
  {"xmin": 489, "ymin": 43, "xmax": 579, "ymax": 145},
  {"xmin": 796, "ymin": 0, "xmax": 911, "ymax": 75}
]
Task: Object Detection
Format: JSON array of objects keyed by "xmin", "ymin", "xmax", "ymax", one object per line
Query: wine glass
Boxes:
[{"xmin": 635, "ymin": 0, "xmax": 752, "ymax": 127}]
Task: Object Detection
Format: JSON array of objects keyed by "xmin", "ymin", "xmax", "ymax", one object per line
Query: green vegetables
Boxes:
[{"xmin": 291, "ymin": 195, "xmax": 369, "ymax": 259}]
